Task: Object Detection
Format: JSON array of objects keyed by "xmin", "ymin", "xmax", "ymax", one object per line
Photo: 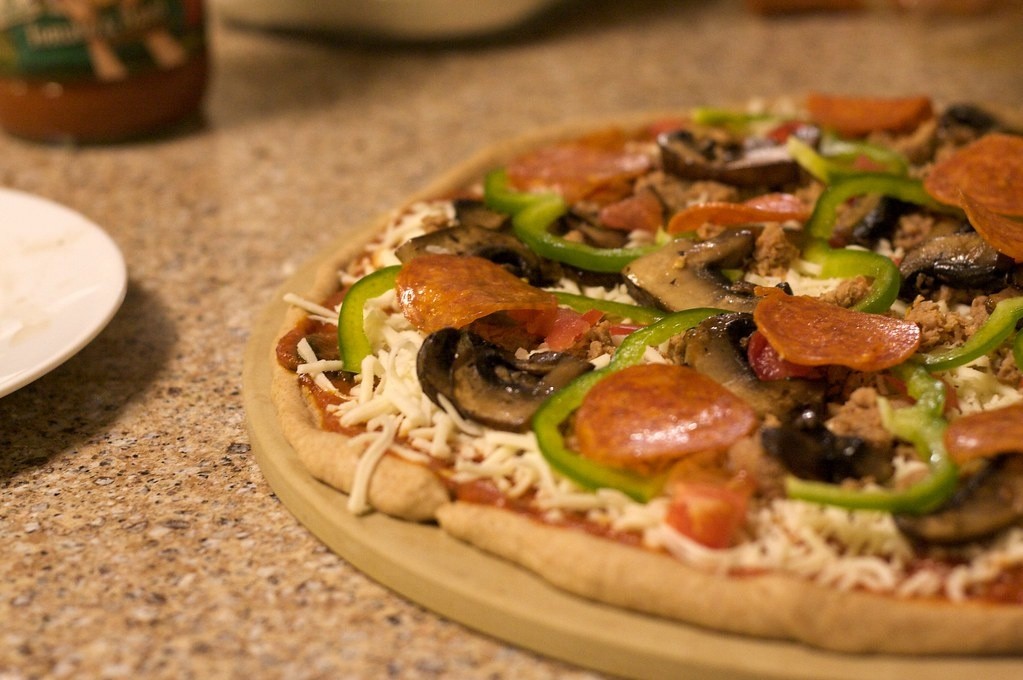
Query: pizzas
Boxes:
[{"xmin": 267, "ymin": 92, "xmax": 1023, "ymax": 657}]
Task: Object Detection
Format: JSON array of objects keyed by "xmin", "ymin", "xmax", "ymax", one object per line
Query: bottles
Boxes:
[{"xmin": 0, "ymin": 0, "xmax": 206, "ymax": 144}]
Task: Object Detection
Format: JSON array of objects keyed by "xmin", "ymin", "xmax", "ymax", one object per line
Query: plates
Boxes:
[{"xmin": 0, "ymin": 184, "xmax": 128, "ymax": 400}]
